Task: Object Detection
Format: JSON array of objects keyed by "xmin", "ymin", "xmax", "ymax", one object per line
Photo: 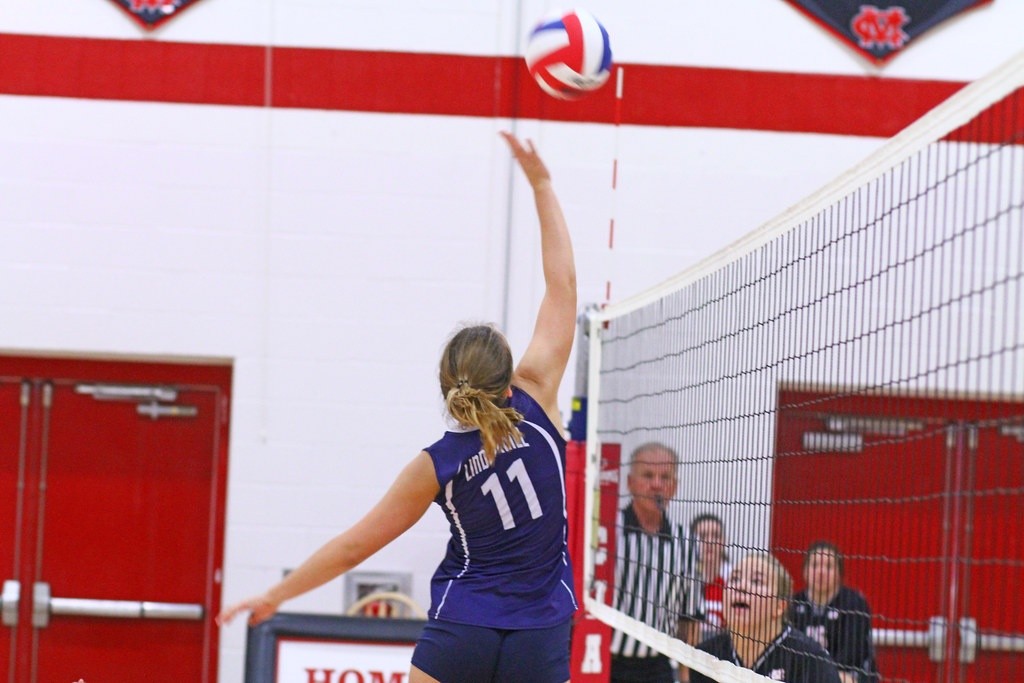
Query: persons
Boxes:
[
  {"xmin": 689, "ymin": 553, "xmax": 843, "ymax": 683},
  {"xmin": 608, "ymin": 440, "xmax": 708, "ymax": 683},
  {"xmin": 685, "ymin": 515, "xmax": 739, "ymax": 641},
  {"xmin": 214, "ymin": 130, "xmax": 578, "ymax": 683},
  {"xmin": 787, "ymin": 540, "xmax": 881, "ymax": 683}
]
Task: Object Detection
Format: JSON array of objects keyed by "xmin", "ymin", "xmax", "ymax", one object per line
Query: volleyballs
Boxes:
[{"xmin": 521, "ymin": 3, "xmax": 612, "ymax": 102}]
